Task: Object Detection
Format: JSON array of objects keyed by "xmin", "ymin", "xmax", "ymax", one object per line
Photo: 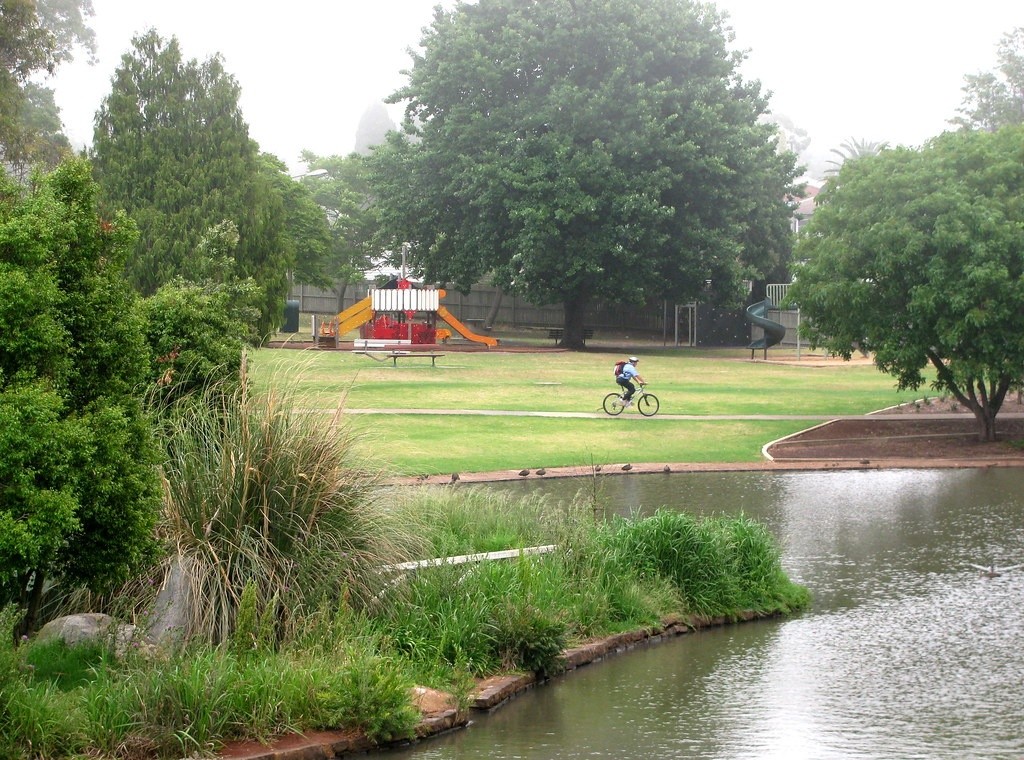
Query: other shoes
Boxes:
[
  {"xmin": 626, "ymin": 402, "xmax": 631, "ymax": 408},
  {"xmin": 618, "ymin": 398, "xmax": 627, "ymax": 406}
]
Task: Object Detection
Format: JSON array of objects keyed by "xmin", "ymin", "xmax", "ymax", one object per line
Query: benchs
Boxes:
[
  {"xmin": 384, "ymin": 344, "xmax": 446, "ymax": 366},
  {"xmin": 547, "ymin": 329, "xmax": 595, "ymax": 349},
  {"xmin": 351, "ymin": 339, "xmax": 411, "ymax": 361}
]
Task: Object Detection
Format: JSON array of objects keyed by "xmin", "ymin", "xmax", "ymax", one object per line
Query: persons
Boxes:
[{"xmin": 616, "ymin": 357, "xmax": 650, "ymax": 407}]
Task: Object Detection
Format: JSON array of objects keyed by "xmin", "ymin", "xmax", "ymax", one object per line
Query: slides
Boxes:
[{"xmin": 437, "ymin": 304, "xmax": 498, "ymax": 348}]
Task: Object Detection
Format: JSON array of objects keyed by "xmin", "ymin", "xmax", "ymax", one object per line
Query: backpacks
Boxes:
[{"xmin": 614, "ymin": 361, "xmax": 630, "ymax": 377}]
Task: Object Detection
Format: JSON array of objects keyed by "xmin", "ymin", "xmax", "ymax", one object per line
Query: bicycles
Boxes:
[{"xmin": 603, "ymin": 382, "xmax": 660, "ymax": 416}]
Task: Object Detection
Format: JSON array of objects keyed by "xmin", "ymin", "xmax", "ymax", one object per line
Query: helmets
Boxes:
[{"xmin": 629, "ymin": 356, "xmax": 639, "ymax": 362}]
